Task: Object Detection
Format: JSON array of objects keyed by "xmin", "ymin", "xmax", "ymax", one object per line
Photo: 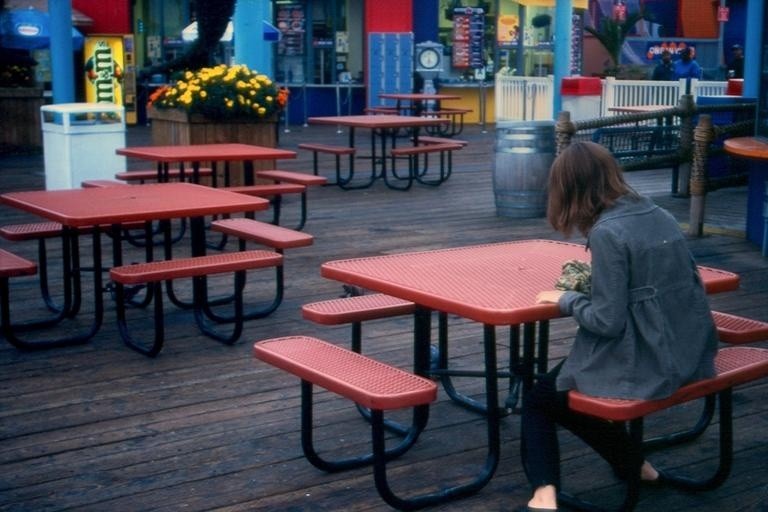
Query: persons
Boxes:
[
  {"xmin": 650, "ymin": 48, "xmax": 675, "ymax": 81},
  {"xmin": 513, "ymin": 140, "xmax": 718, "ymax": 512},
  {"xmin": 673, "ymin": 46, "xmax": 702, "ymax": 80},
  {"xmin": 726, "ymin": 44, "xmax": 745, "ymax": 80}
]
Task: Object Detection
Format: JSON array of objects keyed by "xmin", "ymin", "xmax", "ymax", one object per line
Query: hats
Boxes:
[
  {"xmin": 676, "ymin": 48, "xmax": 689, "ymax": 52},
  {"xmin": 731, "ymin": 44, "xmax": 741, "ymax": 49}
]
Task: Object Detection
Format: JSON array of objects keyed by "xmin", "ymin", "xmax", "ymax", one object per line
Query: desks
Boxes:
[
  {"xmin": 380, "ymin": 91, "xmax": 464, "ymax": 114},
  {"xmin": 310, "ymin": 114, "xmax": 449, "ymax": 180},
  {"xmin": 116, "ymin": 142, "xmax": 296, "ymax": 247},
  {"xmin": 608, "ymin": 102, "xmax": 674, "ymax": 115},
  {"xmin": 2, "ymin": 180, "xmax": 270, "ymax": 315},
  {"xmin": 319, "ymin": 238, "xmax": 741, "ymax": 459}
]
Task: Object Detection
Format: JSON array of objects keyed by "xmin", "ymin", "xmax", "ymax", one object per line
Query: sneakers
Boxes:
[
  {"xmin": 506, "ymin": 504, "xmax": 563, "ymax": 512},
  {"xmin": 638, "ymin": 470, "xmax": 670, "ymax": 485}
]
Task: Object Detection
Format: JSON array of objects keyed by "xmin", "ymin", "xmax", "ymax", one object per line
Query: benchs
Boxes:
[
  {"xmin": 393, "ymin": 145, "xmax": 463, "ymax": 191},
  {"xmin": 422, "ymin": 110, "xmax": 471, "ymax": 114},
  {"xmin": 254, "ymin": 334, "xmax": 438, "ymax": 512},
  {"xmin": 366, "ymin": 107, "xmax": 397, "ymax": 113},
  {"xmin": 379, "ymin": 104, "xmax": 417, "ymax": 108},
  {"xmin": 81, "ymin": 180, "xmax": 126, "ymax": 187},
  {"xmin": 411, "ymin": 135, "xmax": 468, "ymax": 180},
  {"xmin": 117, "ymin": 166, "xmax": 215, "ymax": 181},
  {"xmin": 299, "ymin": 142, "xmax": 356, "ymax": 190},
  {"xmin": 1, "ymin": 235, "xmax": 38, "ymax": 352},
  {"xmin": 566, "ymin": 346, "xmax": 768, "ymax": 512},
  {"xmin": 586, "ymin": 121, "xmax": 687, "ymax": 195},
  {"xmin": 302, "ymin": 294, "xmax": 469, "ymax": 351},
  {"xmin": 0, "ymin": 222, "xmax": 156, "ymax": 313},
  {"xmin": 109, "ymin": 248, "xmax": 284, "ymax": 358},
  {"xmin": 437, "ymin": 106, "xmax": 475, "ymax": 111},
  {"xmin": 221, "ymin": 167, "xmax": 328, "ymax": 228},
  {"xmin": 209, "ymin": 218, "xmax": 315, "ymax": 313},
  {"xmin": 692, "ymin": 303, "xmax": 768, "ymax": 425}
]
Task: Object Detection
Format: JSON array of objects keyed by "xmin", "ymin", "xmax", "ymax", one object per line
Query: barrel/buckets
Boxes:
[{"xmin": 490, "ymin": 122, "xmax": 557, "ymax": 218}]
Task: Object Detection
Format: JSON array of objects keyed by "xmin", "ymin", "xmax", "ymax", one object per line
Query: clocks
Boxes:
[{"xmin": 418, "ymin": 49, "xmax": 441, "ymax": 70}]
